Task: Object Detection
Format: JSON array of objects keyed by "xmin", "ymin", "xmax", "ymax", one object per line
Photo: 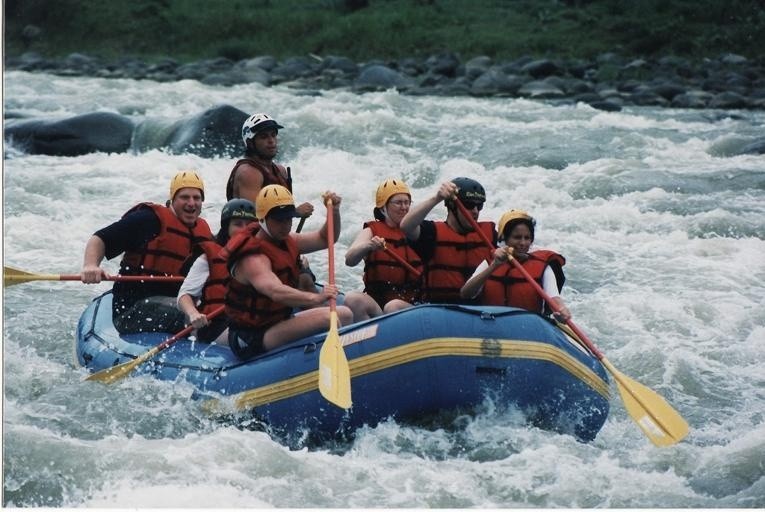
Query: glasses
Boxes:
[
  {"xmin": 392, "ymin": 200, "xmax": 413, "ymax": 206},
  {"xmin": 463, "ymin": 200, "xmax": 483, "ymax": 210}
]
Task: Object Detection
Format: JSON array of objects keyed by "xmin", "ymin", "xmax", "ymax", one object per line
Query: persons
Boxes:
[
  {"xmin": 337, "ymin": 177, "xmax": 424, "ymax": 323},
  {"xmin": 225, "ymin": 113, "xmax": 292, "ymax": 203},
  {"xmin": 173, "ymin": 196, "xmax": 257, "ymax": 343},
  {"xmin": 226, "ymin": 180, "xmax": 353, "ymax": 361},
  {"xmin": 452, "ymin": 211, "xmax": 571, "ymax": 326},
  {"xmin": 398, "ymin": 177, "xmax": 499, "ymax": 300},
  {"xmin": 79, "ymin": 170, "xmax": 217, "ymax": 343}
]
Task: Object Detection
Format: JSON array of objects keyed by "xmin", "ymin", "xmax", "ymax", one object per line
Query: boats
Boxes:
[{"xmin": 78, "ymin": 287, "xmax": 609, "ymax": 450}]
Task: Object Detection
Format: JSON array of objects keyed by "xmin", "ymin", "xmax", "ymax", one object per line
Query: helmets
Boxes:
[
  {"xmin": 444, "ymin": 177, "xmax": 486, "ymax": 209},
  {"xmin": 221, "ymin": 197, "xmax": 256, "ymax": 223},
  {"xmin": 242, "ymin": 113, "xmax": 284, "ymax": 148},
  {"xmin": 170, "ymin": 171, "xmax": 205, "ymax": 203},
  {"xmin": 256, "ymin": 183, "xmax": 295, "ymax": 218},
  {"xmin": 376, "ymin": 177, "xmax": 411, "ymax": 208},
  {"xmin": 497, "ymin": 210, "xmax": 536, "ymax": 242}
]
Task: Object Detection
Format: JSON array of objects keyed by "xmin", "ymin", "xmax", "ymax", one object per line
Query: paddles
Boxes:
[
  {"xmin": 83, "ymin": 300, "xmax": 234, "ymax": 393},
  {"xmin": 503, "ymin": 250, "xmax": 691, "ymax": 449},
  {"xmin": 2, "ymin": 264, "xmax": 188, "ymax": 288},
  {"xmin": 318, "ymin": 197, "xmax": 354, "ymax": 411},
  {"xmin": 455, "ymin": 197, "xmax": 591, "ymax": 355}
]
